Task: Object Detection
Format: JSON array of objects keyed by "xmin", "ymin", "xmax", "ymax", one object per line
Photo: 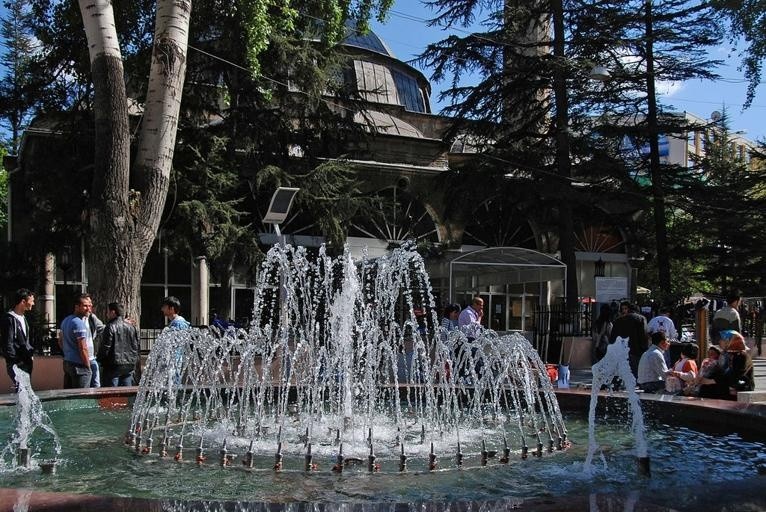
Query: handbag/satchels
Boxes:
[{"xmin": 664, "ymin": 374, "xmax": 681, "ymax": 392}]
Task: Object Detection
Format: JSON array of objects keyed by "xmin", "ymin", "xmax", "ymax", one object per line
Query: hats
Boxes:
[
  {"xmin": 710, "ymin": 345, "xmax": 724, "ymax": 355},
  {"xmin": 721, "ymin": 330, "xmax": 733, "ymax": 340}
]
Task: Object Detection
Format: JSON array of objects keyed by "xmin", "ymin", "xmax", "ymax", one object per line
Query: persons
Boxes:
[
  {"xmin": 57, "ymin": 292, "xmax": 191, "ymax": 387},
  {"xmin": 709, "ymin": 293, "xmax": 741, "ymax": 345},
  {"xmin": 592, "ymin": 300, "xmax": 678, "ymax": 393},
  {"xmin": 458, "ymin": 297, "xmax": 485, "ymax": 379},
  {"xmin": 1, "ymin": 289, "xmax": 33, "ymax": 393},
  {"xmin": 669, "ymin": 330, "xmax": 756, "ymax": 401},
  {"xmin": 441, "ymin": 303, "xmax": 461, "ymax": 379}
]
